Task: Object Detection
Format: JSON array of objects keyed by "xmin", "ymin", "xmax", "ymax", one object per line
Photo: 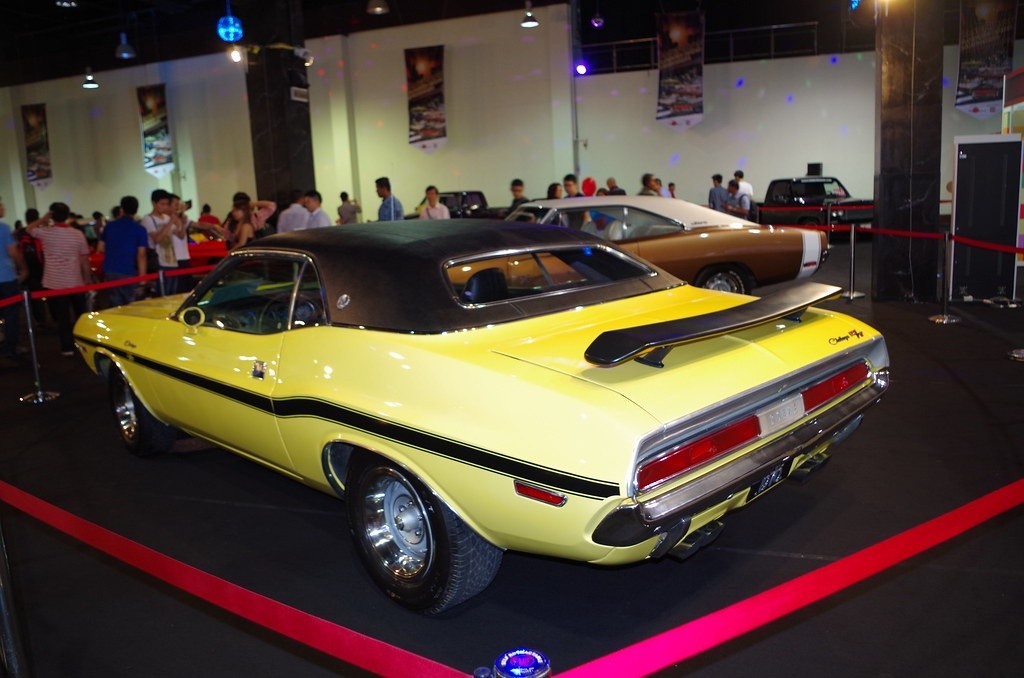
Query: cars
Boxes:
[
  {"xmin": 763, "ymin": 176, "xmax": 875, "ymax": 232},
  {"xmin": 445, "ymin": 197, "xmax": 830, "ymax": 302},
  {"xmin": 71, "ymin": 220, "xmax": 892, "ymax": 616},
  {"xmin": 23, "ymin": 224, "xmax": 230, "ymax": 275}
]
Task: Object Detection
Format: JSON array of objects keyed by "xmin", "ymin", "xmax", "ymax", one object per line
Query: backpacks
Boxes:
[
  {"xmin": 738, "ymin": 194, "xmax": 757, "ymax": 221},
  {"xmin": 17, "ymin": 236, "xmax": 43, "ymax": 276}
]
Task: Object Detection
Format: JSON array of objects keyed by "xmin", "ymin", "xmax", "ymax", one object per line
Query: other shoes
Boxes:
[{"xmin": 60, "ymin": 348, "xmax": 74, "ymax": 356}]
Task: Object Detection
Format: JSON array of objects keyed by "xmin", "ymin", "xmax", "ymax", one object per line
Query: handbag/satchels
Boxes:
[{"xmin": 147, "ymin": 248, "xmax": 159, "ymax": 272}]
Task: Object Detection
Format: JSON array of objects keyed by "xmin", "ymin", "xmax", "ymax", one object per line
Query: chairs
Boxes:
[
  {"xmin": 460, "ymin": 267, "xmax": 509, "ymax": 304},
  {"xmin": 603, "ymin": 220, "xmax": 623, "ymax": 240},
  {"xmin": 645, "ymin": 224, "xmax": 682, "ymax": 235}
]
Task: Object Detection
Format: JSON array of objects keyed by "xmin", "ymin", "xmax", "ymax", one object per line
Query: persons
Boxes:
[
  {"xmin": 375, "ymin": 176, "xmax": 404, "ymax": 221},
  {"xmin": 506, "ymin": 171, "xmax": 754, "ymax": 240},
  {"xmin": 0, "ymin": 188, "xmax": 332, "ymax": 356},
  {"xmin": 337, "ymin": 191, "xmax": 362, "ymax": 223},
  {"xmin": 420, "ymin": 185, "xmax": 451, "ymax": 219}
]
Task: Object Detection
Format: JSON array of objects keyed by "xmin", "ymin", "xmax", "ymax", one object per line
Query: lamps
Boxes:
[
  {"xmin": 215, "ymin": 0, "xmax": 244, "ymax": 44},
  {"xmin": 365, "ymin": 0, "xmax": 390, "ymax": 15},
  {"xmin": 81, "ymin": 67, "xmax": 100, "ymax": 90},
  {"xmin": 519, "ymin": 0, "xmax": 540, "ymax": 29},
  {"xmin": 113, "ymin": 33, "xmax": 137, "ymax": 60}
]
